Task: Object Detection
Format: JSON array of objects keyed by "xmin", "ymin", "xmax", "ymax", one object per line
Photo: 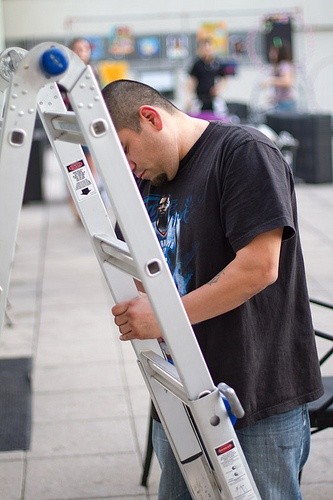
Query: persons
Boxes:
[
  {"xmin": 98, "ymin": 23, "xmax": 299, "ymax": 117},
  {"xmin": 88, "ymin": 79, "xmax": 321, "ymax": 500},
  {"xmin": 56, "ymin": 36, "xmax": 103, "ymax": 226}
]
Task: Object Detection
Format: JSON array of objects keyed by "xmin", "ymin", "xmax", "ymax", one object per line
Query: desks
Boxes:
[{"xmin": 267, "ymin": 114, "xmax": 332, "ymax": 183}]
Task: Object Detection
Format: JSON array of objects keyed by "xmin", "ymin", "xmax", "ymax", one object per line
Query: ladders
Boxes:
[{"xmin": 0, "ymin": 41, "xmax": 263, "ymax": 500}]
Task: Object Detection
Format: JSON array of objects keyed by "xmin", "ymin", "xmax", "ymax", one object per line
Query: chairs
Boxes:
[{"xmin": 141, "ymin": 297, "xmax": 333, "ymax": 487}]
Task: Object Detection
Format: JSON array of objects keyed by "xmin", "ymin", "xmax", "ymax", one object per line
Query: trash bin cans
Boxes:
[{"xmin": 275, "ymin": 131, "xmax": 300, "ymax": 172}]
